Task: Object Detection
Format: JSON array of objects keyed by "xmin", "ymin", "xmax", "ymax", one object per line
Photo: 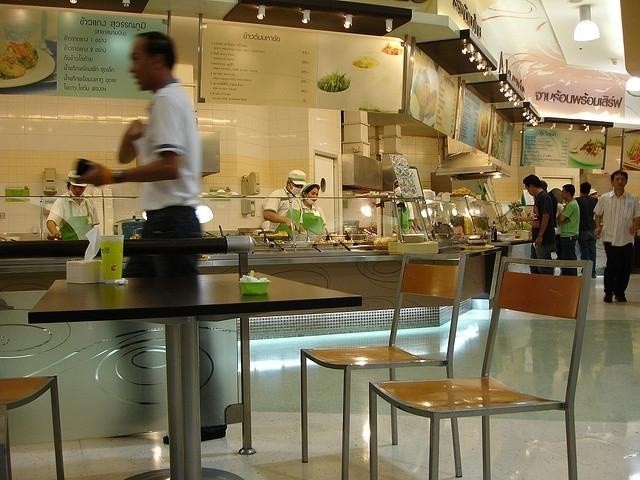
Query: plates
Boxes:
[
  {"xmin": 317, "ymin": 76, "xmax": 351, "ymax": 94},
  {"xmin": 0, "ymin": 48, "xmax": 56, "ymax": 88},
  {"xmin": 478, "ymin": 111, "xmax": 490, "ymax": 148},
  {"xmin": 351, "ymin": 60, "xmax": 377, "ymax": 70},
  {"xmin": 568, "ymin": 135, "xmax": 603, "ymax": 166}
]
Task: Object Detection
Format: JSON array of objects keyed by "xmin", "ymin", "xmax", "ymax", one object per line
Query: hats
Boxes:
[
  {"xmin": 287, "ymin": 169, "xmax": 307, "ymax": 186},
  {"xmin": 67, "ymin": 168, "xmax": 88, "ymax": 187},
  {"xmin": 300, "ymin": 182, "xmax": 320, "ymax": 194},
  {"xmin": 587, "ymin": 188, "xmax": 598, "ymax": 197}
]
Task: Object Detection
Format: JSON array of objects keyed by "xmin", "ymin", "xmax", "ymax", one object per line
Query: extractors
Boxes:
[{"xmin": 435, "ymin": 137, "xmax": 512, "ymax": 181}]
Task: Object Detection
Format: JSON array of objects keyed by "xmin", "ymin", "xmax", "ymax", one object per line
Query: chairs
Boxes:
[
  {"xmin": 0, "ymin": 376, "xmax": 64, "ymax": 480},
  {"xmin": 368, "ymin": 256, "xmax": 593, "ymax": 480},
  {"xmin": 300, "ymin": 254, "xmax": 465, "ymax": 480}
]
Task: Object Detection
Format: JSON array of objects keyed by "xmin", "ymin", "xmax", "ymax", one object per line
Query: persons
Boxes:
[
  {"xmin": 529, "ymin": 303, "xmax": 640, "ymax": 437},
  {"xmin": 593, "ymin": 170, "xmax": 640, "ymax": 302},
  {"xmin": 79, "ymin": 31, "xmax": 228, "ymax": 442},
  {"xmin": 46, "ymin": 169, "xmax": 100, "ymax": 239},
  {"xmin": 260, "ymin": 169, "xmax": 306, "ymax": 237},
  {"xmin": 523, "ymin": 174, "xmax": 599, "ymax": 278},
  {"xmin": 299, "ymin": 182, "xmax": 329, "ymax": 237}
]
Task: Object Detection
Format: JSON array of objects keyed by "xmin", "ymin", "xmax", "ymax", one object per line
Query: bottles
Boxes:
[{"xmin": 491, "ymin": 221, "xmax": 498, "ymax": 242}]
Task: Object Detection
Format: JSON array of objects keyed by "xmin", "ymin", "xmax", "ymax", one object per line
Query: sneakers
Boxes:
[
  {"xmin": 615, "ymin": 292, "xmax": 627, "ymax": 302},
  {"xmin": 603, "ymin": 293, "xmax": 613, "ymax": 303},
  {"xmin": 630, "ymin": 232, "xmax": 640, "ymax": 274}
]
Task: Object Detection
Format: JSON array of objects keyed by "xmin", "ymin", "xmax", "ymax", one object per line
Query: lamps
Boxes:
[
  {"xmin": 256, "ymin": 4, "xmax": 393, "ymax": 33},
  {"xmin": 573, "ymin": 4, "xmax": 601, "ymax": 42}
]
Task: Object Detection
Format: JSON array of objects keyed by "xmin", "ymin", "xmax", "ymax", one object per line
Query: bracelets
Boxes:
[{"xmin": 112, "ymin": 169, "xmax": 123, "ymax": 182}]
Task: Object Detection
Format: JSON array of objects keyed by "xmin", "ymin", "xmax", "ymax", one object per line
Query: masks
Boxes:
[
  {"xmin": 288, "ymin": 181, "xmax": 301, "ymax": 196},
  {"xmin": 306, "ymin": 198, "xmax": 319, "ymax": 205}
]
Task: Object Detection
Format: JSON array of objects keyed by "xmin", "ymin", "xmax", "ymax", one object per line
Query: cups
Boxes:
[{"xmin": 99, "ymin": 234, "xmax": 124, "ymax": 283}]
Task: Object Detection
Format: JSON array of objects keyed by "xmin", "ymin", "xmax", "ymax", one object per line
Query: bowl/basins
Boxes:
[
  {"xmin": 374, "ymin": 236, "xmax": 398, "ymax": 250},
  {"xmin": 239, "ymin": 281, "xmax": 270, "ymax": 296}
]
